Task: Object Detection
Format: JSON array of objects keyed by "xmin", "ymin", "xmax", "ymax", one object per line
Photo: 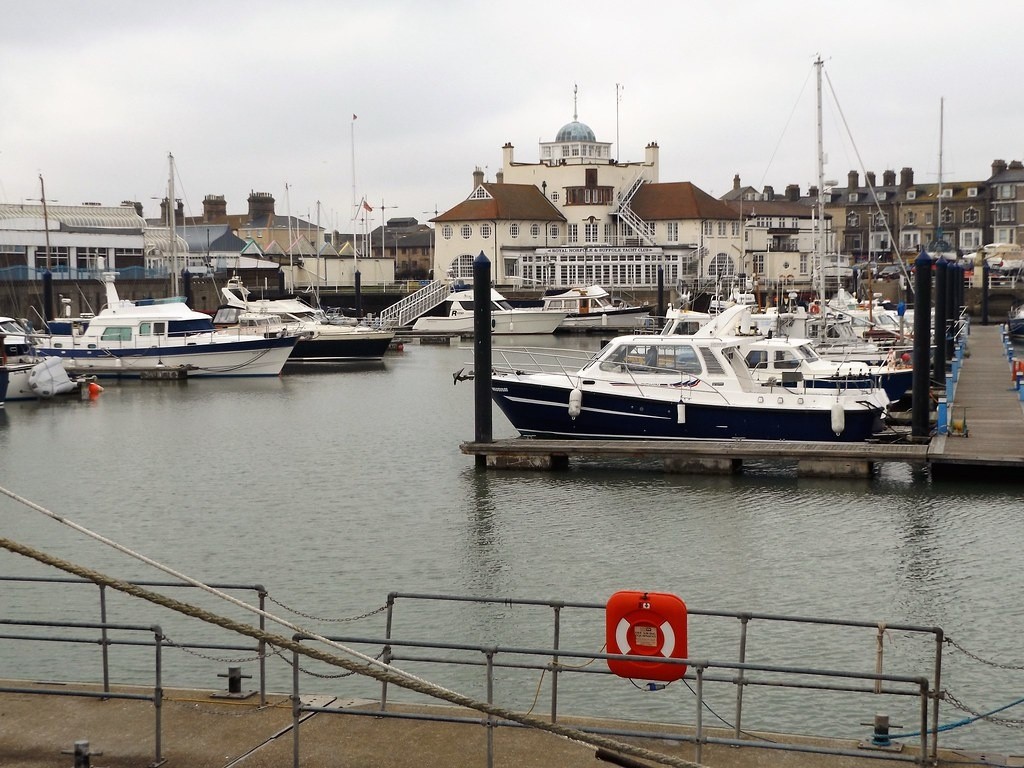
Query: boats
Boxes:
[{"xmin": 1, "ymin": 47, "xmax": 935, "ymax": 442}]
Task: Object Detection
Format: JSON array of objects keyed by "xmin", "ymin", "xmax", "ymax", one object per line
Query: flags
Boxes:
[{"xmin": 364, "ymin": 201, "xmax": 372, "ymax": 212}]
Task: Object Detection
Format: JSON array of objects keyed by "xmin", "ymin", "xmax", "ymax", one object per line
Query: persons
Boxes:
[{"xmin": 810, "ymin": 301, "xmax": 820, "ymax": 336}]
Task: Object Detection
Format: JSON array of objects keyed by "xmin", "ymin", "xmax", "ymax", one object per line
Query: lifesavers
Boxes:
[
  {"xmin": 787, "ymin": 274, "xmax": 794, "ymax": 282},
  {"xmin": 810, "ymin": 305, "xmax": 819, "ymax": 315},
  {"xmin": 886, "ymin": 353, "xmax": 894, "ymax": 366},
  {"xmin": 779, "ymin": 275, "xmax": 786, "ymax": 282}
]
